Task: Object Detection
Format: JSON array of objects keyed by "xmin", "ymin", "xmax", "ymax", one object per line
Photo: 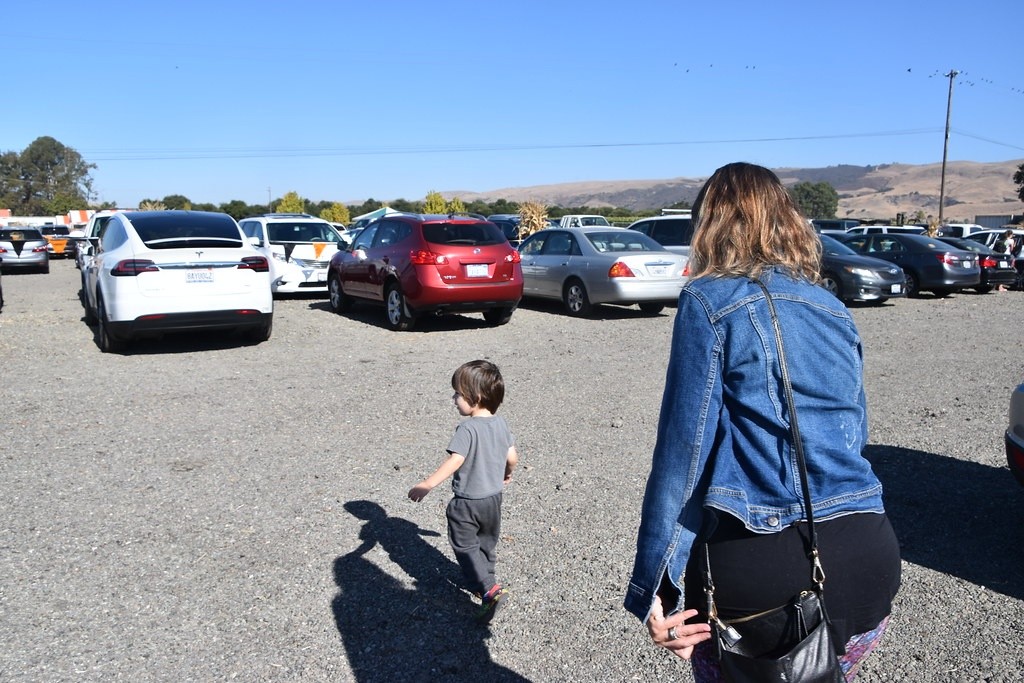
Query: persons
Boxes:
[
  {"xmin": 995, "ymin": 229, "xmax": 1016, "ymax": 292},
  {"xmin": 409, "ymin": 358, "xmax": 520, "ymax": 617},
  {"xmin": 624, "ymin": 162, "xmax": 901, "ymax": 683},
  {"xmin": 923, "ymin": 214, "xmax": 946, "ymax": 239}
]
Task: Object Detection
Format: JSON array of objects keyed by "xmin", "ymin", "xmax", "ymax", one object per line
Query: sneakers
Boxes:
[{"xmin": 477, "ymin": 584, "xmax": 508, "ymax": 625}]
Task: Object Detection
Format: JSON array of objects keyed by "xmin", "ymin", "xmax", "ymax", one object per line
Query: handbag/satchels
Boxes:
[{"xmin": 712, "ymin": 582, "xmax": 848, "ymax": 683}]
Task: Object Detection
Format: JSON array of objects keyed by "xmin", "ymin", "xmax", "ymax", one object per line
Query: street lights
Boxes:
[{"xmin": 938, "ymin": 69, "xmax": 961, "ymax": 226}]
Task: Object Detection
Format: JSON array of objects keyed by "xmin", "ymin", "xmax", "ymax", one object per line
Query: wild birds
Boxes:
[
  {"xmin": 674, "ymin": 62, "xmax": 712, "ymax": 73},
  {"xmin": 746, "ymin": 66, "xmax": 755, "ymax": 68},
  {"xmin": 907, "ymin": 67, "xmax": 1023, "ymax": 93}
]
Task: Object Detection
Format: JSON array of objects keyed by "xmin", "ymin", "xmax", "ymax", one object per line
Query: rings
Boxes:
[{"xmin": 668, "ymin": 624, "xmax": 679, "ymax": 641}]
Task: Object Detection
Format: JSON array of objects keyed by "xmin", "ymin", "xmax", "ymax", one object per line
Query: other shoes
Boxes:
[{"xmin": 999, "ymin": 288, "xmax": 1007, "ymax": 291}]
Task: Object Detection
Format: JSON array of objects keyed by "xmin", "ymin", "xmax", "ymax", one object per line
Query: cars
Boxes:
[
  {"xmin": 813, "ymin": 233, "xmax": 906, "ymax": 305},
  {"xmin": 886, "ymin": 237, "xmax": 1019, "ymax": 294},
  {"xmin": 0, "ymin": 226, "xmax": 50, "ymax": 274},
  {"xmin": 516, "ymin": 226, "xmax": 691, "ymax": 318},
  {"xmin": 825, "ymin": 233, "xmax": 980, "ymax": 299},
  {"xmin": 38, "ymin": 225, "xmax": 78, "ymax": 260},
  {"xmin": 329, "ymin": 222, "xmax": 391, "ymax": 244}
]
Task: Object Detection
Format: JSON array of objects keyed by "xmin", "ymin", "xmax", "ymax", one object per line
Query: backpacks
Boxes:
[{"xmin": 993, "ymin": 239, "xmax": 1009, "ymax": 253}]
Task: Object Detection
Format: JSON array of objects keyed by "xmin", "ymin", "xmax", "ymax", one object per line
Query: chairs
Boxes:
[
  {"xmin": 627, "ymin": 243, "xmax": 642, "ymax": 250},
  {"xmin": 872, "ymin": 242, "xmax": 883, "ymax": 251},
  {"xmin": 549, "ymin": 236, "xmax": 570, "ymax": 254},
  {"xmin": 890, "ymin": 243, "xmax": 901, "ymax": 251},
  {"xmin": 596, "ymin": 242, "xmax": 606, "ymax": 250}
]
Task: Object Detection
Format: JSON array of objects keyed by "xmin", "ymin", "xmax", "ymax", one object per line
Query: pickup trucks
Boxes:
[{"xmin": 558, "ymin": 215, "xmax": 616, "ymax": 242}]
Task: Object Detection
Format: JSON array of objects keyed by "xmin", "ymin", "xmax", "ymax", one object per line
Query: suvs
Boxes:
[
  {"xmin": 75, "ymin": 211, "xmax": 132, "ymax": 270},
  {"xmin": 486, "ymin": 214, "xmax": 569, "ymax": 251},
  {"xmin": 610, "ymin": 209, "xmax": 693, "ymax": 256},
  {"xmin": 959, "ymin": 229, "xmax": 1024, "ymax": 291},
  {"xmin": 805, "ymin": 218, "xmax": 985, "ymax": 254},
  {"xmin": 82, "ymin": 209, "xmax": 273, "ymax": 354},
  {"xmin": 237, "ymin": 213, "xmax": 345, "ymax": 293},
  {"xmin": 326, "ymin": 210, "xmax": 524, "ymax": 332}
]
